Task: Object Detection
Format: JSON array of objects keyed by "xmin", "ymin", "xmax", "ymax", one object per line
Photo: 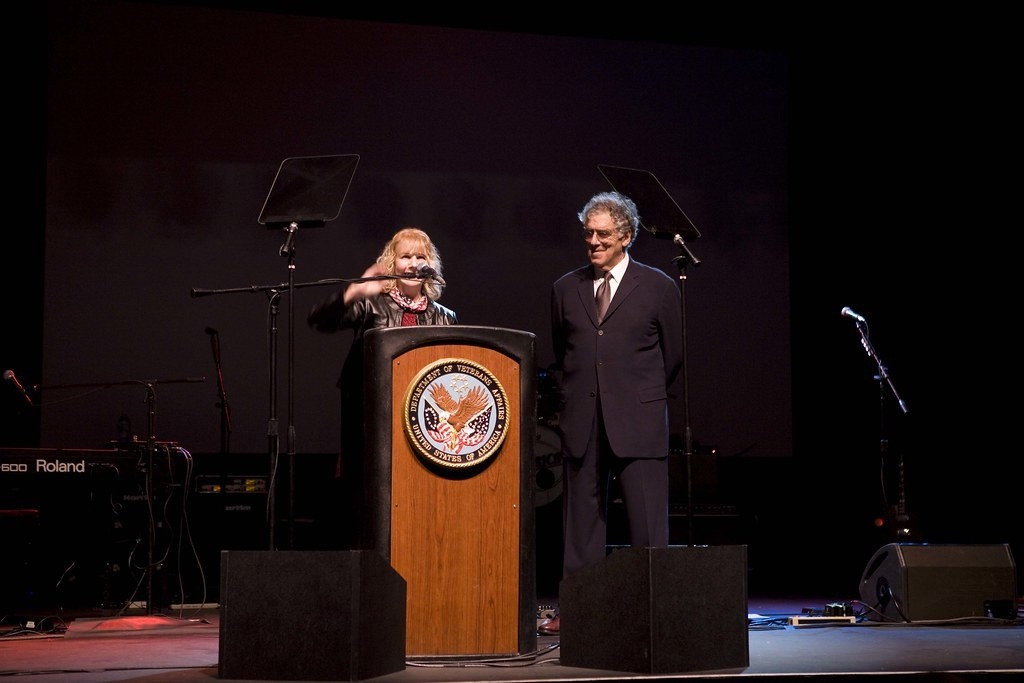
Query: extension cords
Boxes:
[{"xmin": 787, "ymin": 615, "xmax": 856, "ymax": 627}]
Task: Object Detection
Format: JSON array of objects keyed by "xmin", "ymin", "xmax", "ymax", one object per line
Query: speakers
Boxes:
[
  {"xmin": 559, "ymin": 444, "xmax": 753, "ymax": 675},
  {"xmin": 857, "ymin": 544, "xmax": 1021, "ymax": 623},
  {"xmin": 218, "ymin": 550, "xmax": 407, "ymax": 682}
]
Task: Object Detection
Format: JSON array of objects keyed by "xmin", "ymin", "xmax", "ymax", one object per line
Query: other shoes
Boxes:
[{"xmin": 536, "ymin": 614, "xmax": 560, "ymax": 635}]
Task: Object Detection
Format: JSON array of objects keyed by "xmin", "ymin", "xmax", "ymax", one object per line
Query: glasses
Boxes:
[{"xmin": 581, "ymin": 229, "xmax": 620, "ymax": 242}]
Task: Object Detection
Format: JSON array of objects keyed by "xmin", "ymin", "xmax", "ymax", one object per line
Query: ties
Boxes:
[{"xmin": 595, "ymin": 271, "xmax": 614, "ymax": 326}]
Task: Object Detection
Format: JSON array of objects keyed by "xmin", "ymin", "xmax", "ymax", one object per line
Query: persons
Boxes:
[
  {"xmin": 308, "ymin": 228, "xmax": 458, "ymax": 550},
  {"xmin": 537, "ymin": 191, "xmax": 682, "ymax": 637}
]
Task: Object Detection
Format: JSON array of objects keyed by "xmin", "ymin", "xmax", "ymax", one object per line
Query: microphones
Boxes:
[
  {"xmin": 841, "ymin": 307, "xmax": 865, "ymax": 322},
  {"xmin": 3, "ymin": 370, "xmax": 32, "ymax": 404},
  {"xmin": 417, "ymin": 261, "xmax": 446, "ymax": 286},
  {"xmin": 204, "ymin": 327, "xmax": 219, "ymax": 336}
]
